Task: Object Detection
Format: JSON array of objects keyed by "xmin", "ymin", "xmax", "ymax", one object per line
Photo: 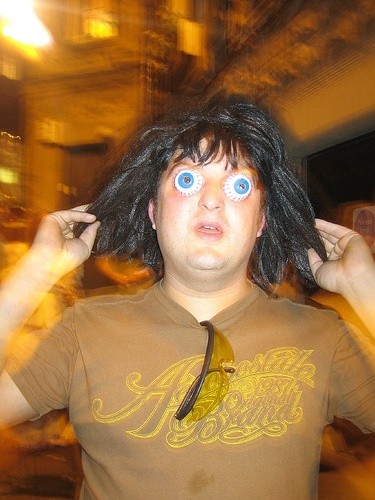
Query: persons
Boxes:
[{"xmin": 0, "ymin": 90, "xmax": 375, "ymax": 500}]
